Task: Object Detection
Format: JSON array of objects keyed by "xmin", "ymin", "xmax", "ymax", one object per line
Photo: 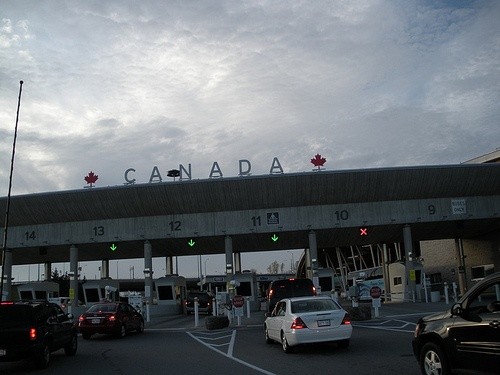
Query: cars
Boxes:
[
  {"xmin": 410, "ymin": 272, "xmax": 500, "ymax": 375},
  {"xmin": 263, "ymin": 296, "xmax": 353, "ymax": 354},
  {"xmin": 78, "ymin": 302, "xmax": 144, "ymax": 340}
]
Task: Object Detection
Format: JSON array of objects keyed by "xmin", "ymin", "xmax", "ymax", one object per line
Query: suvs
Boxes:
[
  {"xmin": 184, "ymin": 292, "xmax": 216, "ymax": 315},
  {"xmin": 0, "ymin": 300, "xmax": 80, "ymax": 372}
]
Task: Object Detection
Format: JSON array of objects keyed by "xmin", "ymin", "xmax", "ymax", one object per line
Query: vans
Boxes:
[{"xmin": 265, "ymin": 278, "xmax": 318, "ymax": 318}]
